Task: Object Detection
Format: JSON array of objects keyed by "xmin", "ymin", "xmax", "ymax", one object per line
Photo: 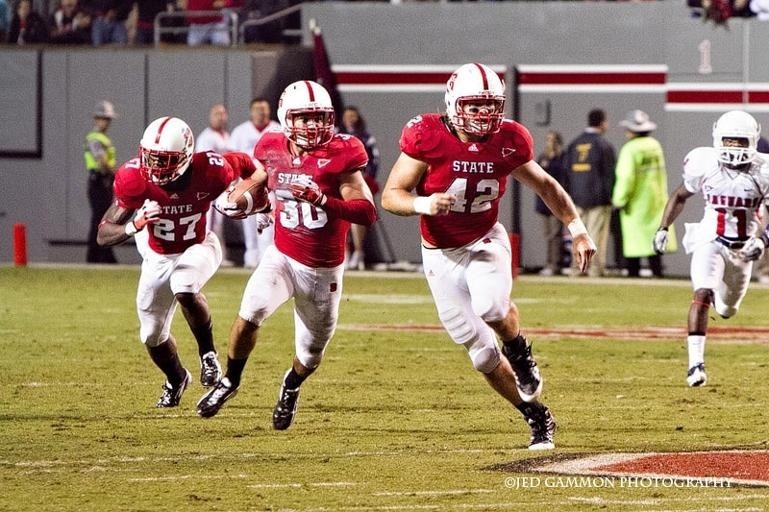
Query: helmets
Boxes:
[
  {"xmin": 712, "ymin": 110, "xmax": 761, "ymax": 168},
  {"xmin": 444, "ymin": 59, "xmax": 507, "ymax": 138},
  {"xmin": 276, "ymin": 77, "xmax": 336, "ymax": 149},
  {"xmin": 138, "ymin": 115, "xmax": 195, "ymax": 187}
]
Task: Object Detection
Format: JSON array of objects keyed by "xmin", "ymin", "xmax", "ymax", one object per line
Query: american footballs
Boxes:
[{"xmin": 227, "ymin": 180, "xmax": 270, "ymax": 216}]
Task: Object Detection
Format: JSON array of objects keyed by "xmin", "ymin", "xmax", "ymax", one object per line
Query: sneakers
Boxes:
[
  {"xmin": 157, "ymin": 368, "xmax": 191, "ymax": 408},
  {"xmin": 522, "ymin": 406, "xmax": 556, "ymax": 450},
  {"xmin": 199, "ymin": 349, "xmax": 222, "ymax": 387},
  {"xmin": 500, "ymin": 335, "xmax": 542, "ymax": 403},
  {"xmin": 196, "ymin": 376, "xmax": 240, "ymax": 419},
  {"xmin": 272, "ymin": 368, "xmax": 300, "ymax": 431},
  {"xmin": 686, "ymin": 362, "xmax": 706, "ymax": 388}
]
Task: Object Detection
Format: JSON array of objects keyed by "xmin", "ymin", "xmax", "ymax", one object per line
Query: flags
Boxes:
[{"xmin": 311, "ymin": 26, "xmax": 338, "ymax": 121}]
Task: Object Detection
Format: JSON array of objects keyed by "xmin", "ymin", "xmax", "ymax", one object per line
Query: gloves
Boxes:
[
  {"xmin": 216, "ymin": 183, "xmax": 276, "ymax": 234},
  {"xmin": 286, "ymin": 178, "xmax": 324, "ymax": 207},
  {"xmin": 126, "ymin": 196, "xmax": 164, "ymax": 239},
  {"xmin": 739, "ymin": 238, "xmax": 765, "ymax": 263},
  {"xmin": 652, "ymin": 226, "xmax": 668, "ymax": 257}
]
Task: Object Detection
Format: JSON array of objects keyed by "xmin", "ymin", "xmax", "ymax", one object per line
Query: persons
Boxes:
[
  {"xmin": 0, "ymin": 0, "xmax": 296, "ymax": 47},
  {"xmin": 85, "ymin": 63, "xmax": 768, "ymax": 450}
]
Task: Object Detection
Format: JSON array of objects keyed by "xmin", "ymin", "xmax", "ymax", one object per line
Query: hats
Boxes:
[
  {"xmin": 618, "ymin": 107, "xmax": 658, "ymax": 134},
  {"xmin": 93, "ymin": 100, "xmax": 118, "ymax": 121}
]
